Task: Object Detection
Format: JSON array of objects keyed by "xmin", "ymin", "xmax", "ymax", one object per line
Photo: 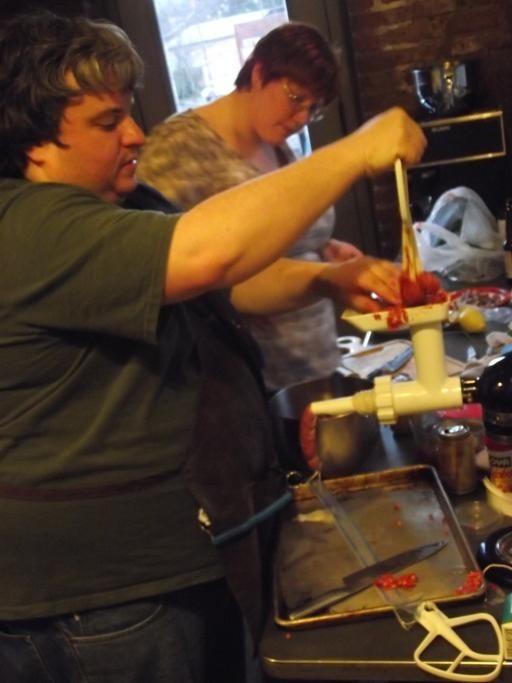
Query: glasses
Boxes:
[{"xmin": 280, "ymin": 78, "xmax": 325, "ymax": 122}]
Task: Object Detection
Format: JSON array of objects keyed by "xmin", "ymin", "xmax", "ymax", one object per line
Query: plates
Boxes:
[{"xmin": 444, "ymin": 286, "xmax": 510, "ymax": 312}]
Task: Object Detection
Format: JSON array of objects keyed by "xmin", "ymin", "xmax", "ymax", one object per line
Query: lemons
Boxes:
[{"xmin": 458, "ymin": 307, "xmax": 485, "ymax": 333}]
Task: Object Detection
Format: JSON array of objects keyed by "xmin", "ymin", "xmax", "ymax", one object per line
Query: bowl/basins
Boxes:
[{"xmin": 264, "ymin": 374, "xmax": 378, "ymax": 477}]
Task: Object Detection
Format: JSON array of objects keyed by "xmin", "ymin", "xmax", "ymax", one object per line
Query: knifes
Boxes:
[{"xmin": 286, "ymin": 539, "xmax": 451, "ymax": 622}]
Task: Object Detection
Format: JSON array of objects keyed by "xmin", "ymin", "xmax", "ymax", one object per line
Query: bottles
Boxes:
[
  {"xmin": 475, "ymin": 350, "xmax": 512, "ymax": 492},
  {"xmin": 500, "ymin": 198, "xmax": 512, "ymax": 284}
]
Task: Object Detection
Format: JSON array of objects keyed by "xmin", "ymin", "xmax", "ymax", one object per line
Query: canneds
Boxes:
[{"xmin": 434, "ymin": 422, "xmax": 478, "ymax": 499}]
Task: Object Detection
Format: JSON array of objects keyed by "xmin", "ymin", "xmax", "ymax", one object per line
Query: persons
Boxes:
[
  {"xmin": 1, "ymin": 4, "xmax": 429, "ymax": 680},
  {"xmin": 134, "ymin": 18, "xmax": 390, "ymax": 392}
]
